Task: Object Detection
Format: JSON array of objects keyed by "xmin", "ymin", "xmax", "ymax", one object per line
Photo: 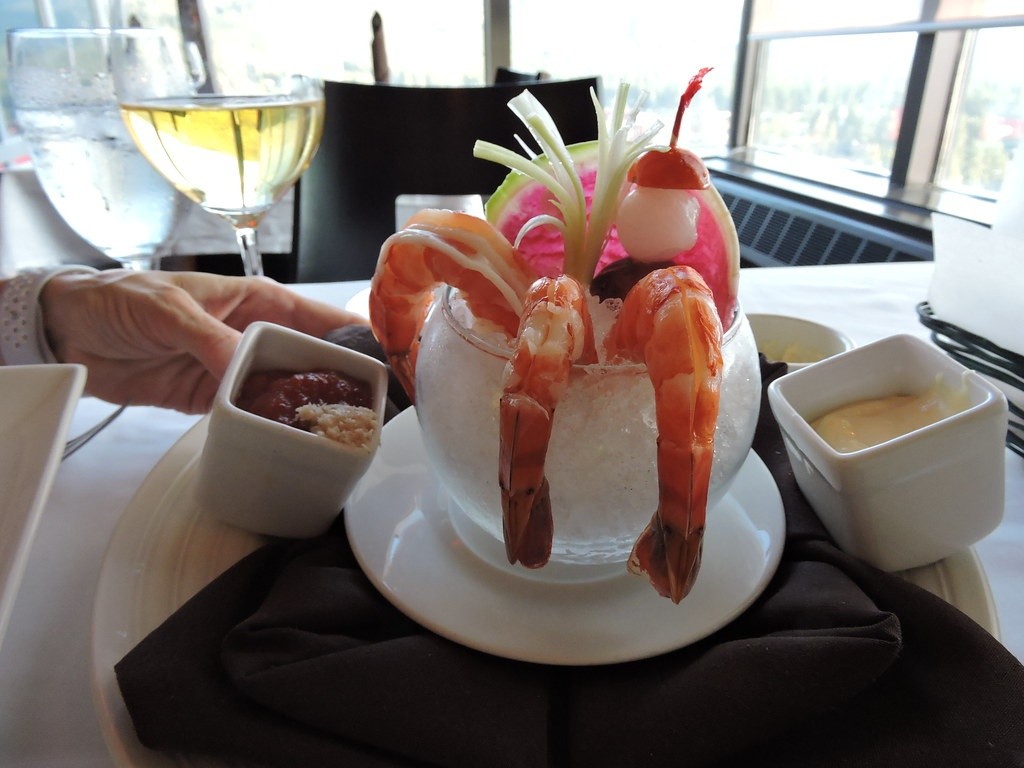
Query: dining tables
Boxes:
[{"xmin": 0, "ymin": 258, "xmax": 1024, "ymax": 767}]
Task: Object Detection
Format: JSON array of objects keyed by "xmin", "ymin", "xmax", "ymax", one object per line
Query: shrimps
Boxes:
[{"xmin": 367, "ymin": 208, "xmax": 719, "ymax": 605}]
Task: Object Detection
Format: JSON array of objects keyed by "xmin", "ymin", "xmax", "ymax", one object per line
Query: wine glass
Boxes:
[
  {"xmin": 107, "ymin": 1, "xmax": 325, "ymax": 276},
  {"xmin": 7, "ymin": 26, "xmax": 203, "ymax": 271}
]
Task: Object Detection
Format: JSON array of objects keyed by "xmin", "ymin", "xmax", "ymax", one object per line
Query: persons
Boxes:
[{"xmin": 0, "ymin": 260, "xmax": 379, "ymax": 415}]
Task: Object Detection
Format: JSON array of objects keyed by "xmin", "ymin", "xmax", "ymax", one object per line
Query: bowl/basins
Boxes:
[
  {"xmin": 767, "ymin": 335, "xmax": 1008, "ymax": 574},
  {"xmin": 192, "ymin": 317, "xmax": 389, "ymax": 539},
  {"xmin": 414, "ymin": 279, "xmax": 761, "ymax": 564},
  {"xmin": 745, "ymin": 313, "xmax": 852, "ymax": 373}
]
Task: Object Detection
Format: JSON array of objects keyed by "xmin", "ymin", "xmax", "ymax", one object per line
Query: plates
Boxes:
[
  {"xmin": 91, "ymin": 404, "xmax": 1000, "ymax": 767},
  {"xmin": 342, "ymin": 407, "xmax": 787, "ymax": 665}
]
